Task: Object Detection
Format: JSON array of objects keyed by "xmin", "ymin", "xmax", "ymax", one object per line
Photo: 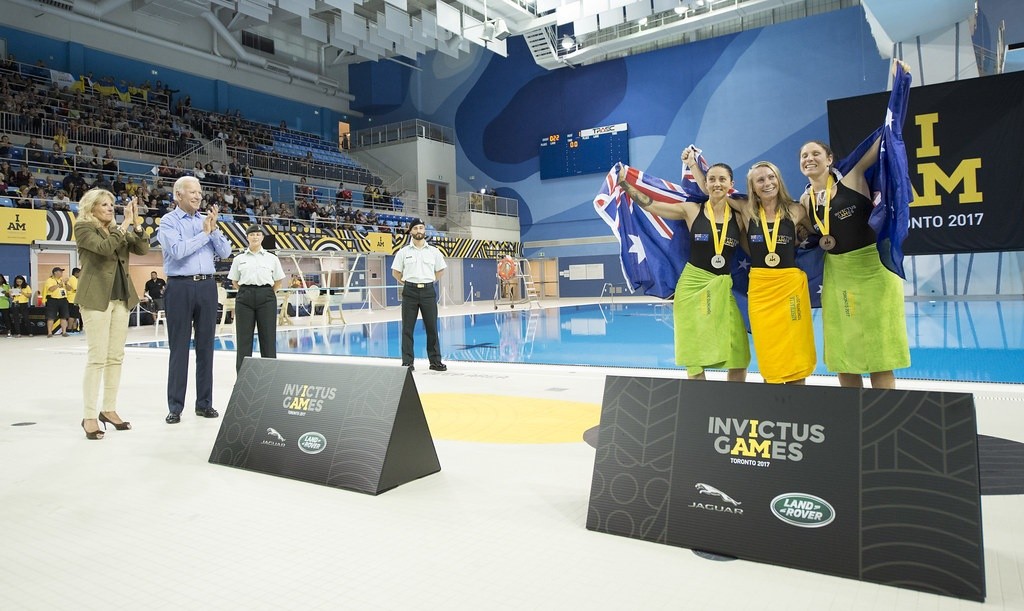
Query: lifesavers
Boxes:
[
  {"xmin": 501, "ymin": 341, "xmax": 517, "ymax": 360},
  {"xmin": 497, "ymin": 258, "xmax": 515, "ymax": 280}
]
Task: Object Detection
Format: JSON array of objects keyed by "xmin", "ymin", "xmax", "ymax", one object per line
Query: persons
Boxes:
[
  {"xmin": 680, "ymin": 145, "xmax": 817, "ymax": 383},
  {"xmin": 144, "ymin": 271, "xmax": 166, "ymax": 323},
  {"xmin": 617, "ymin": 162, "xmax": 753, "ymax": 380},
  {"xmin": 469, "ymin": 187, "xmax": 498, "ymax": 215},
  {"xmin": 155, "ymin": 176, "xmax": 232, "ymax": 424},
  {"xmin": 178, "ymin": 94, "xmax": 314, "ymax": 179},
  {"xmin": 0, "ymin": 52, "xmax": 195, "ymax": 155},
  {"xmin": 427, "ymin": 195, "xmax": 436, "ymax": 216},
  {"xmin": 0, "ymin": 157, "xmax": 411, "ymax": 233},
  {"xmin": 0, "ymin": 126, "xmax": 122, "ymax": 169},
  {"xmin": 42, "ymin": 267, "xmax": 81, "ymax": 337},
  {"xmin": 392, "ymin": 219, "xmax": 448, "ymax": 372},
  {"xmin": 226, "ymin": 224, "xmax": 287, "ymax": 375},
  {"xmin": 71, "ymin": 188, "xmax": 150, "ymax": 439},
  {"xmin": 343, "ymin": 134, "xmax": 348, "ymax": 148},
  {"xmin": 799, "ymin": 58, "xmax": 911, "ymax": 391},
  {"xmin": 0, "ymin": 274, "xmax": 33, "ymax": 337}
]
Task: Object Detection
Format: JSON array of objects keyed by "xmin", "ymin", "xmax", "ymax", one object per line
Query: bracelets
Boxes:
[
  {"xmin": 132, "ymin": 224, "xmax": 143, "ymax": 230},
  {"xmin": 689, "ymin": 163, "xmax": 697, "ymax": 168},
  {"xmin": 116, "ymin": 226, "xmax": 126, "ymax": 235}
]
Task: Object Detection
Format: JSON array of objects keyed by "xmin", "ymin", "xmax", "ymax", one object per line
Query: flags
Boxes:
[{"xmin": 592, "ymin": 62, "xmax": 914, "ymax": 333}]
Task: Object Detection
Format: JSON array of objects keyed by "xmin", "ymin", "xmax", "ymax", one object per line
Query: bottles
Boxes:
[{"xmin": 37, "ymin": 296, "xmax": 42, "ymax": 306}]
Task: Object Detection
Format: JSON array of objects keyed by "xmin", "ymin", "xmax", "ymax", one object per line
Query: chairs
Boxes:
[{"xmin": 1, "ymin": 80, "xmax": 446, "ymax": 237}]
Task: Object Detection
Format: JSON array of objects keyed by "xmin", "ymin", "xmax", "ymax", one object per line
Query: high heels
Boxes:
[
  {"xmin": 99, "ymin": 411, "xmax": 132, "ymax": 430},
  {"xmin": 81, "ymin": 419, "xmax": 105, "ymax": 440}
]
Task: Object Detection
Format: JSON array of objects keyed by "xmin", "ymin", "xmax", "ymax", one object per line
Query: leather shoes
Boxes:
[
  {"xmin": 166, "ymin": 411, "xmax": 181, "ymax": 423},
  {"xmin": 195, "ymin": 407, "xmax": 218, "ymax": 417}
]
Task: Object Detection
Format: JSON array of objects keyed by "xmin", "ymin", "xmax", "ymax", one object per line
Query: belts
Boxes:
[
  {"xmin": 406, "ymin": 282, "xmax": 432, "ymax": 288},
  {"xmin": 168, "ymin": 274, "xmax": 214, "ymax": 281}
]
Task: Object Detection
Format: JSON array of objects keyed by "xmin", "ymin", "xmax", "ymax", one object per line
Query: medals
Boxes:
[
  {"xmin": 765, "ymin": 252, "xmax": 781, "ymax": 267},
  {"xmin": 711, "ymin": 254, "xmax": 725, "ymax": 269},
  {"xmin": 819, "ymin": 235, "xmax": 836, "ymax": 251}
]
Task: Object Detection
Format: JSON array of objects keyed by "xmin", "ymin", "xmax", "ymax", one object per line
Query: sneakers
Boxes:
[
  {"xmin": 430, "ymin": 361, "xmax": 446, "ymax": 370},
  {"xmin": 403, "ymin": 362, "xmax": 414, "ymax": 370}
]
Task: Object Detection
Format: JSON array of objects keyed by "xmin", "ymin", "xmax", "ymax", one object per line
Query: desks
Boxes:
[{"xmin": 0, "ymin": 302, "xmax": 156, "ymax": 334}]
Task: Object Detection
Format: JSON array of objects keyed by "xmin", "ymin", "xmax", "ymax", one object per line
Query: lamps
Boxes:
[
  {"xmin": 480, "ymin": 21, "xmax": 494, "ymax": 42},
  {"xmin": 494, "ymin": 16, "xmax": 512, "ymax": 41},
  {"xmin": 562, "ymin": 38, "xmax": 574, "ymax": 49}
]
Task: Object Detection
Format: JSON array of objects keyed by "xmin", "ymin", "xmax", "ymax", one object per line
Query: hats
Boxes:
[
  {"xmin": 404, "ymin": 218, "xmax": 424, "ymax": 235},
  {"xmin": 52, "ymin": 267, "xmax": 65, "ymax": 272},
  {"xmin": 246, "ymin": 225, "xmax": 262, "ymax": 234}
]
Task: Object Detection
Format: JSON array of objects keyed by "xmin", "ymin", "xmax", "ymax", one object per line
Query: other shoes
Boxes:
[
  {"xmin": 62, "ymin": 333, "xmax": 70, "ymax": 337},
  {"xmin": 16, "ymin": 333, "xmax": 21, "ymax": 338},
  {"xmin": 6, "ymin": 332, "xmax": 11, "ymax": 338},
  {"xmin": 47, "ymin": 334, "xmax": 54, "ymax": 338},
  {"xmin": 29, "ymin": 334, "xmax": 33, "ymax": 337}
]
